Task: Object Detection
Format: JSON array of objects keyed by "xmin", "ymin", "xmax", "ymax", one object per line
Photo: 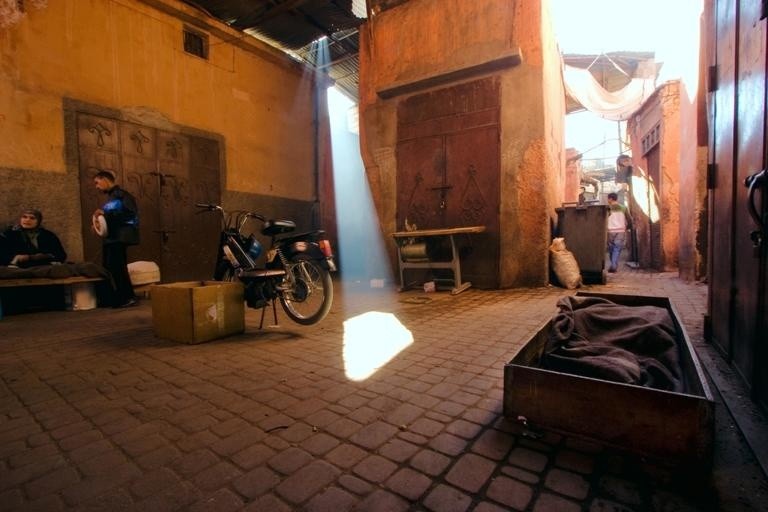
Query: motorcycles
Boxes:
[{"xmin": 195, "ymin": 201, "xmax": 338, "ymax": 330}]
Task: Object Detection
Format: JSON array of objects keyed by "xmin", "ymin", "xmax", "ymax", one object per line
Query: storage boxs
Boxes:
[{"xmin": 150, "ymin": 280, "xmax": 244, "ymax": 345}]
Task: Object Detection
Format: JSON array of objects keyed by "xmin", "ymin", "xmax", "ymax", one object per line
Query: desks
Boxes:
[{"xmin": 386, "ymin": 226, "xmax": 486, "ymax": 295}]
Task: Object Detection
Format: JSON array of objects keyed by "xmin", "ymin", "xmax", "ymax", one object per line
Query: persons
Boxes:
[
  {"xmin": 605, "ymin": 192, "xmax": 633, "ymax": 273},
  {"xmin": 93, "ymin": 171, "xmax": 141, "ymax": 308},
  {"xmin": 0, "ymin": 208, "xmax": 67, "ymax": 314}
]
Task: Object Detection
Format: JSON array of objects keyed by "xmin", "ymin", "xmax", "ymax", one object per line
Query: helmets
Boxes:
[{"xmin": 244, "ymin": 233, "xmax": 262, "ymax": 260}]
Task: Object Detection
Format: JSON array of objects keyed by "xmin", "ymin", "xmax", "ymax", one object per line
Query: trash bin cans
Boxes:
[{"xmin": 555, "ymin": 205, "xmax": 611, "ymax": 285}]
